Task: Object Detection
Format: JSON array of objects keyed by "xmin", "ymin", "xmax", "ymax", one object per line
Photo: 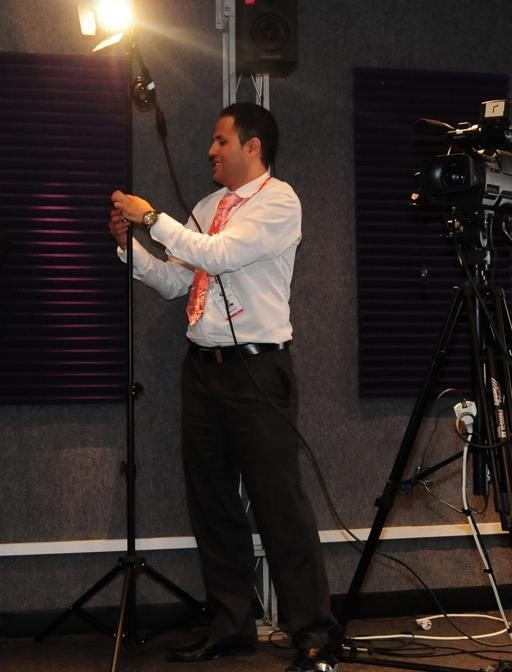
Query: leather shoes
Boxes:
[
  {"xmin": 164, "ymin": 628, "xmax": 259, "ymax": 662},
  {"xmin": 283, "ymin": 646, "xmax": 340, "ymax": 672}
]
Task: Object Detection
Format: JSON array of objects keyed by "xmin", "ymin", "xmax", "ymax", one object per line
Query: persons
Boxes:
[{"xmin": 106, "ymin": 102, "xmax": 340, "ymax": 671}]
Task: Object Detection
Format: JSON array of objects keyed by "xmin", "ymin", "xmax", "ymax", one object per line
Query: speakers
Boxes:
[{"xmin": 235, "ymin": 1, "xmax": 298, "ymax": 76}]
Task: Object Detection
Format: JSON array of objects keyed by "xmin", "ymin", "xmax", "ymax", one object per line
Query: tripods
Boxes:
[
  {"xmin": 31, "ymin": 38, "xmax": 203, "ymax": 672},
  {"xmin": 338, "ymin": 244, "xmax": 511, "ymax": 638}
]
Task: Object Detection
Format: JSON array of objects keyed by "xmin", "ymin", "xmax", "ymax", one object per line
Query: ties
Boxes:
[{"xmin": 185, "ymin": 191, "xmax": 242, "ymax": 328}]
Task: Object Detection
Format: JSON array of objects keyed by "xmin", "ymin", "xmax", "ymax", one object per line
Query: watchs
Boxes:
[{"xmin": 142, "ymin": 210, "xmax": 163, "ymax": 227}]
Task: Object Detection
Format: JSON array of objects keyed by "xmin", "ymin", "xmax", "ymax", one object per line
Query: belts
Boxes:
[{"xmin": 185, "ymin": 339, "xmax": 294, "ymax": 364}]
